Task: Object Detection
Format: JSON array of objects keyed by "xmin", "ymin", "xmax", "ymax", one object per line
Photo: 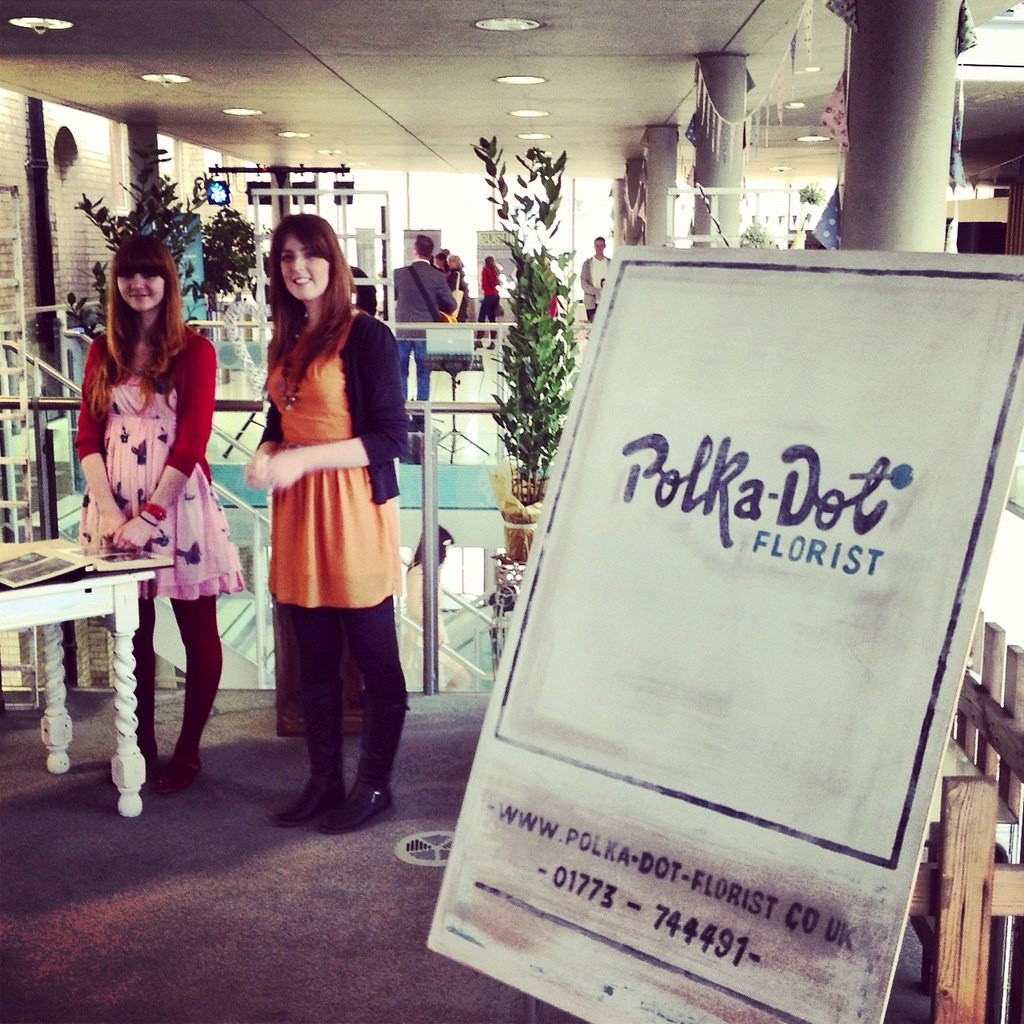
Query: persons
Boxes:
[
  {"xmin": 435, "ymin": 249, "xmax": 469, "ymax": 322},
  {"xmin": 75, "ymin": 234, "xmax": 245, "ymax": 795},
  {"xmin": 581, "ymin": 237, "xmax": 611, "ymax": 323},
  {"xmin": 247, "ymin": 213, "xmax": 410, "ymax": 833},
  {"xmin": 474, "ymin": 256, "xmax": 503, "ymax": 350},
  {"xmin": 394, "ymin": 234, "xmax": 458, "ymax": 401}
]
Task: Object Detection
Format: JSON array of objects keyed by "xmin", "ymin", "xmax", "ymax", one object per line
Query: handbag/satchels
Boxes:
[{"xmin": 450, "ymin": 270, "xmax": 463, "ymax": 318}]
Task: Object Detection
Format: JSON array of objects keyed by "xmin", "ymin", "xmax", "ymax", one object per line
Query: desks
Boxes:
[{"xmin": 0, "ymin": 571, "xmax": 156, "ymax": 818}]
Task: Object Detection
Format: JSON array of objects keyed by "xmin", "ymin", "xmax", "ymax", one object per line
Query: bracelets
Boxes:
[
  {"xmin": 139, "ymin": 515, "xmax": 158, "ymax": 527},
  {"xmin": 144, "ymin": 503, "xmax": 168, "ymax": 522}
]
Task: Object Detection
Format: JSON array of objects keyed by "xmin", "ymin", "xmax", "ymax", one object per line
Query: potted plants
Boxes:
[{"xmin": 472, "ymin": 135, "xmax": 587, "ymax": 568}]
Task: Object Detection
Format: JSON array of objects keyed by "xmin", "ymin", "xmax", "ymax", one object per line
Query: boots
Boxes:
[
  {"xmin": 319, "ymin": 690, "xmax": 409, "ymax": 834},
  {"xmin": 276, "ymin": 690, "xmax": 348, "ymax": 827}
]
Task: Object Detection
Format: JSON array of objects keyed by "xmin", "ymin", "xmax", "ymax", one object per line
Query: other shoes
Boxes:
[
  {"xmin": 475, "ymin": 341, "xmax": 483, "ymax": 348},
  {"xmin": 154, "ymin": 753, "xmax": 200, "ymax": 793},
  {"xmin": 487, "ymin": 342, "xmax": 496, "ymax": 349}
]
